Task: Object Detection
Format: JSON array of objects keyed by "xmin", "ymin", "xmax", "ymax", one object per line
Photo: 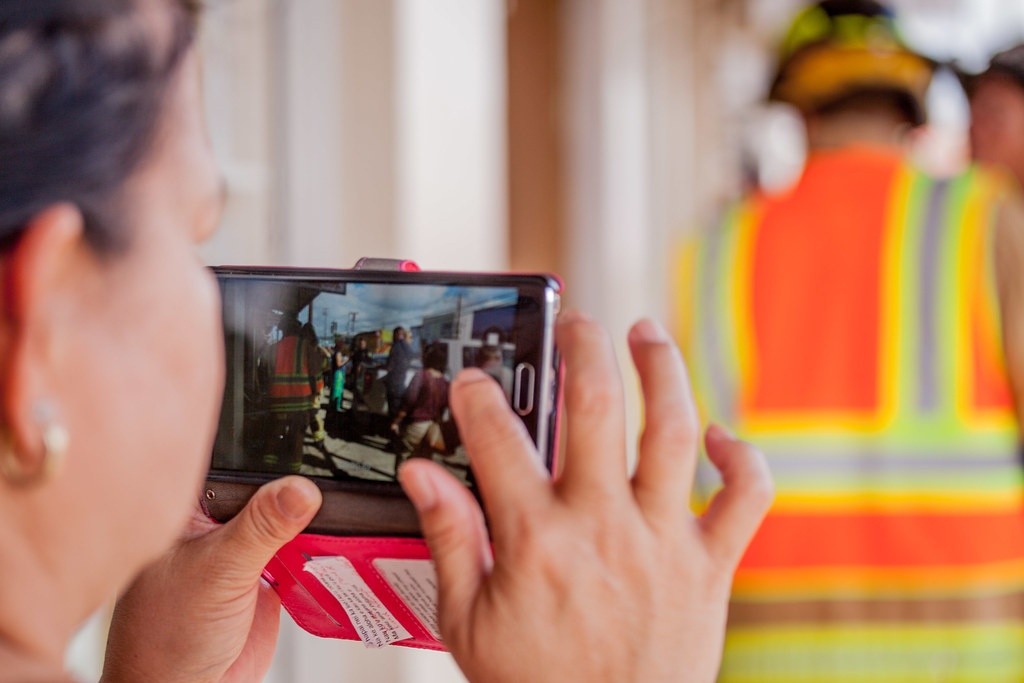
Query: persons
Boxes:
[
  {"xmin": 662, "ymin": 0, "xmax": 1024, "ymax": 683},
  {"xmin": 252, "ymin": 316, "xmax": 513, "ymax": 476},
  {"xmin": 0, "ymin": 0, "xmax": 779, "ymax": 683}
]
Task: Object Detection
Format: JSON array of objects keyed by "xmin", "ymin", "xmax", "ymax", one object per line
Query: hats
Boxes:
[{"xmin": 768, "ymin": 32, "xmax": 940, "ymax": 127}]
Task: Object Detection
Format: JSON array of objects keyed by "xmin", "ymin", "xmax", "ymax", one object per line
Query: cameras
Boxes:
[{"xmin": 193, "ymin": 257, "xmax": 564, "ymax": 539}]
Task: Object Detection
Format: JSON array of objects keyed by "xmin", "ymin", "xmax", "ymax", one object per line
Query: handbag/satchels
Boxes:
[{"xmin": 423, "ymin": 405, "xmax": 457, "ymax": 458}]
